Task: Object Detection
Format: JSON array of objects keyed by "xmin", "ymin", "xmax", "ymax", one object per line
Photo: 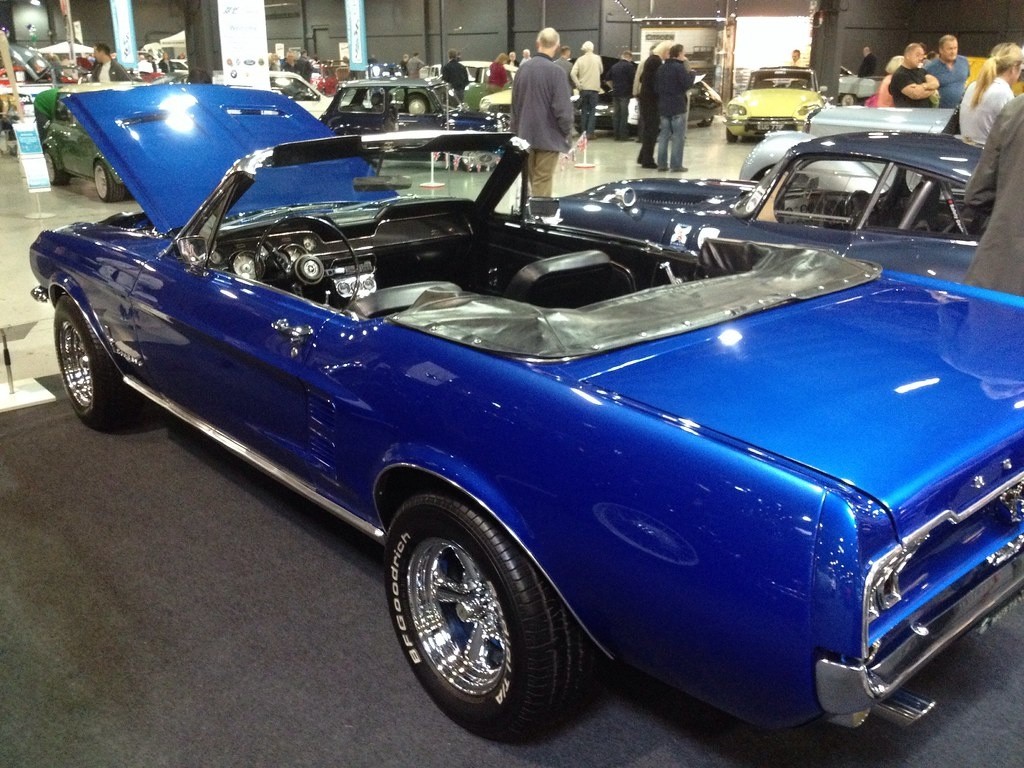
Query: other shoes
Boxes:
[
  {"xmin": 637, "ymin": 159, "xmax": 658, "ymax": 169},
  {"xmin": 657, "ymin": 167, "xmax": 671, "ymax": 172},
  {"xmin": 614, "ymin": 137, "xmax": 634, "ymax": 141},
  {"xmin": 670, "ymin": 167, "xmax": 688, "ymax": 173},
  {"xmin": 587, "ymin": 133, "xmax": 597, "ymax": 139}
]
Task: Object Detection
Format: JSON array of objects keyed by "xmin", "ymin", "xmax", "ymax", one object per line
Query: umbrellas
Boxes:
[{"xmin": 160, "ymin": 31, "xmax": 186, "ymax": 47}]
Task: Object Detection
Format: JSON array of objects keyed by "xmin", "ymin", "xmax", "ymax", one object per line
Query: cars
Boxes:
[
  {"xmin": 29, "ymin": 80, "xmax": 1024, "ymax": 745},
  {"xmin": 33, "ymin": 64, "xmax": 1000, "ymax": 296}
]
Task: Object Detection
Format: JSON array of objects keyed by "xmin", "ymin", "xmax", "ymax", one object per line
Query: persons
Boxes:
[
  {"xmin": 553, "ymin": 41, "xmax": 696, "ymax": 172},
  {"xmin": 487, "ymin": 50, "xmax": 532, "ymax": 87},
  {"xmin": 442, "ymin": 48, "xmax": 469, "ymax": 102},
  {"xmin": 45, "ymin": 43, "xmax": 185, "ymax": 82},
  {"xmin": 963, "ymin": 93, "xmax": 1024, "ymax": 297},
  {"xmin": 268, "ymin": 47, "xmax": 425, "ymax": 97},
  {"xmin": 858, "ymin": 34, "xmax": 1024, "ymax": 147},
  {"xmin": 787, "ymin": 50, "xmax": 806, "ymax": 68},
  {"xmin": 511, "ymin": 27, "xmax": 573, "ymax": 196}
]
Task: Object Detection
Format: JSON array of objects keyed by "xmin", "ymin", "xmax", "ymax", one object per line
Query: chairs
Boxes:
[
  {"xmin": 347, "ymin": 282, "xmax": 463, "ymax": 320},
  {"xmin": 501, "ymin": 250, "xmax": 638, "ymax": 310},
  {"xmin": 758, "ymin": 81, "xmax": 776, "ymax": 88},
  {"xmin": 284, "ymin": 82, "xmax": 301, "ymax": 98},
  {"xmin": 371, "ymin": 93, "xmax": 383, "ymax": 112},
  {"xmin": 789, "ymin": 81, "xmax": 803, "ymax": 88},
  {"xmin": 382, "ymin": 93, "xmax": 396, "ymax": 111}
]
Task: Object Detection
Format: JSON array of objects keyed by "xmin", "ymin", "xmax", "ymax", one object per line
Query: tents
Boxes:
[{"xmin": 37, "ymin": 42, "xmax": 94, "ymax": 60}]
